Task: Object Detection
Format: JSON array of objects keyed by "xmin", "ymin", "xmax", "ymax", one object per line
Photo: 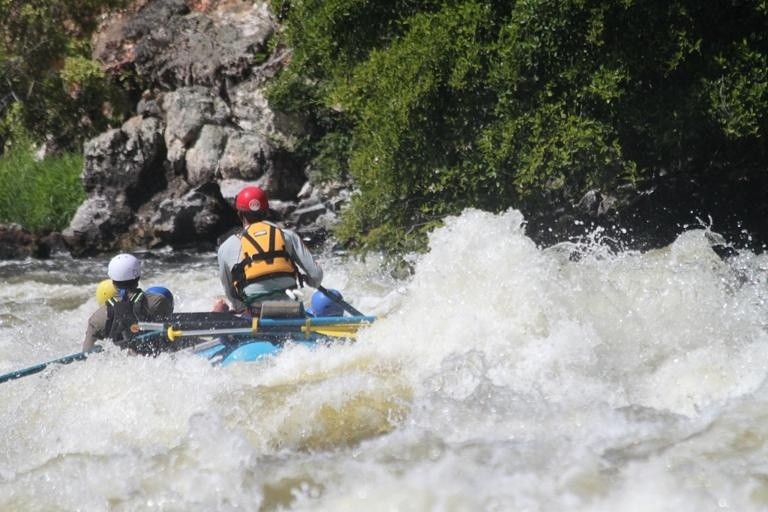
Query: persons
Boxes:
[
  {"xmin": 97, "ymin": 279, "xmax": 119, "ymax": 307},
  {"xmin": 145, "ymin": 287, "xmax": 174, "ymax": 313},
  {"xmin": 82, "ymin": 253, "xmax": 229, "ymax": 353},
  {"xmin": 217, "ymin": 186, "xmax": 323, "ymax": 314}
]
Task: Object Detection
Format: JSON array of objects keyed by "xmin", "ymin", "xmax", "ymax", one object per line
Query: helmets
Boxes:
[
  {"xmin": 147, "ymin": 287, "xmax": 173, "ymax": 311},
  {"xmin": 97, "ymin": 279, "xmax": 118, "ymax": 305},
  {"xmin": 235, "ymin": 187, "xmax": 269, "ymax": 212},
  {"xmin": 108, "ymin": 253, "xmax": 140, "ymax": 281}
]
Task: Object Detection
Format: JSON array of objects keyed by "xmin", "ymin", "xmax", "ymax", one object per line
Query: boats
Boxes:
[{"xmin": 85, "ymin": 303, "xmax": 382, "ymax": 373}]
